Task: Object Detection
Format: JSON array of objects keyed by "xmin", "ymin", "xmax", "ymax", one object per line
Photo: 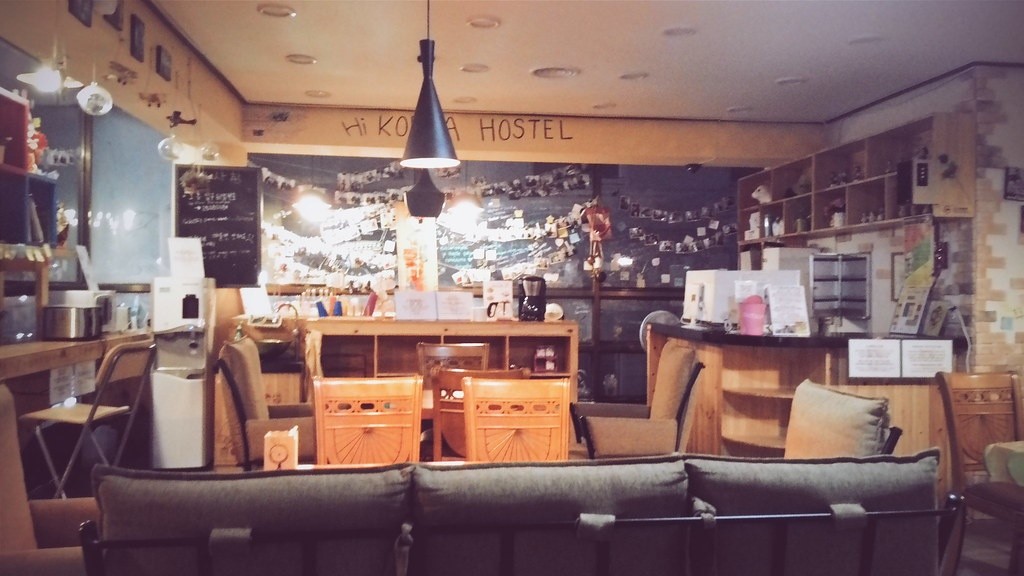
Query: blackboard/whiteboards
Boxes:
[
  {"xmin": 172, "ymin": 163, "xmax": 263, "ymax": 289},
  {"xmin": 247, "ymin": 145, "xmax": 774, "ymax": 296}
]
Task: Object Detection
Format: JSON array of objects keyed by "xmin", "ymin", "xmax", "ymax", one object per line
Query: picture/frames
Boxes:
[{"xmin": 890, "ymin": 251, "xmax": 906, "ymax": 300}]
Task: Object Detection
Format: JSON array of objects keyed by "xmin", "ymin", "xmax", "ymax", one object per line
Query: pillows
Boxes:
[{"xmin": 783, "ymin": 380, "xmax": 884, "ymax": 465}]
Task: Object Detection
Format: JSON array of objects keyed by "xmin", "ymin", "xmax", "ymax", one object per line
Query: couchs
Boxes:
[{"xmin": 79, "ymin": 450, "xmax": 964, "ymax": 576}]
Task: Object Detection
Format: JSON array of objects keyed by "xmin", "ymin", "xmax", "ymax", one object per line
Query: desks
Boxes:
[
  {"xmin": 984, "ymin": 440, "xmax": 1024, "ymax": 488},
  {"xmin": 0, "ymin": 328, "xmax": 154, "ymax": 404},
  {"xmin": 307, "ymin": 319, "xmax": 582, "ymax": 420}
]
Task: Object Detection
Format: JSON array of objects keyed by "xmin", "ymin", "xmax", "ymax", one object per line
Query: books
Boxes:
[
  {"xmin": 764, "ymin": 286, "xmax": 811, "ymax": 336},
  {"xmin": 847, "ymin": 339, "xmax": 953, "ymax": 377}
]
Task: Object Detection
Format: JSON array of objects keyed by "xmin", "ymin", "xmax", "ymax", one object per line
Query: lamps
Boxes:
[
  {"xmin": 400, "ymin": 2, "xmax": 461, "ymax": 169},
  {"xmin": 403, "ymin": 169, "xmax": 444, "ymax": 218}
]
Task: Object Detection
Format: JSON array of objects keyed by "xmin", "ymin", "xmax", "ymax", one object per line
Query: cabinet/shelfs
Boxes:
[
  {"xmin": 738, "ymin": 111, "xmax": 973, "ymax": 247},
  {"xmin": 641, "ymin": 323, "xmax": 969, "ymax": 471}
]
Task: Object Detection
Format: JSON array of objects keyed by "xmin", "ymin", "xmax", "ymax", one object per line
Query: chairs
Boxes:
[
  {"xmin": 0, "ymin": 387, "xmax": 109, "ymax": 576},
  {"xmin": 463, "ymin": 378, "xmax": 570, "ymax": 469},
  {"xmin": 936, "ymin": 369, "xmax": 1024, "ymax": 576},
  {"xmin": 428, "ymin": 367, "xmax": 529, "ymax": 462},
  {"xmin": 417, "ymin": 344, "xmax": 490, "ymax": 394},
  {"xmin": 208, "ymin": 336, "xmax": 320, "ymax": 471},
  {"xmin": 571, "ymin": 341, "xmax": 706, "ymax": 460},
  {"xmin": 312, "ymin": 376, "xmax": 423, "ymax": 465},
  {"xmin": 20, "ymin": 339, "xmax": 155, "ymax": 502}
]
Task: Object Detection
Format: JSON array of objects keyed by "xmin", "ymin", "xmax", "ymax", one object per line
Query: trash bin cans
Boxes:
[{"xmin": 147, "ymin": 367, "xmax": 205, "ymax": 471}]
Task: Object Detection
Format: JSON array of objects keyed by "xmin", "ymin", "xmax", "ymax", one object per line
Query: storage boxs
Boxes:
[{"xmin": 264, "ymin": 427, "xmax": 298, "ymax": 470}]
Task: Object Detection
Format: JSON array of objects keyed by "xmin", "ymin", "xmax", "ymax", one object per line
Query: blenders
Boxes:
[{"xmin": 518, "ymin": 276, "xmax": 547, "ymax": 321}]
